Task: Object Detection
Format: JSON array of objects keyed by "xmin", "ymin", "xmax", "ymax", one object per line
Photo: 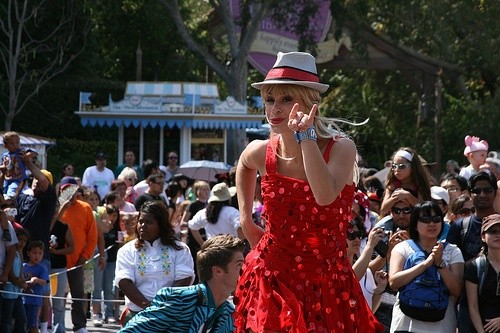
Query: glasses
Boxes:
[
  {"xmin": 443, "ymin": 185, "xmax": 463, "ymax": 192},
  {"xmin": 458, "ymin": 207, "xmax": 476, "ymax": 215},
  {"xmin": 346, "ymin": 230, "xmax": 364, "ymax": 241},
  {"xmin": 169, "ymin": 157, "xmax": 178, "ymax": 159},
  {"xmin": 153, "ymin": 181, "xmax": 165, "ymax": 185},
  {"xmin": 418, "ymin": 215, "xmax": 443, "ymax": 224},
  {"xmin": 391, "ymin": 163, "xmax": 412, "ymax": 169},
  {"xmin": 392, "ymin": 206, "xmax": 412, "ymax": 214},
  {"xmin": 470, "ymin": 187, "xmax": 493, "ymax": 194}
]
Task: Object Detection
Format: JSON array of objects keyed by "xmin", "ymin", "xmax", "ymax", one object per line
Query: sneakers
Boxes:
[
  {"xmin": 75, "ymin": 327, "xmax": 89, "ymax": 333},
  {"xmin": 106, "ymin": 314, "xmax": 116, "ymax": 323},
  {"xmin": 93, "ymin": 314, "xmax": 103, "ymax": 326}
]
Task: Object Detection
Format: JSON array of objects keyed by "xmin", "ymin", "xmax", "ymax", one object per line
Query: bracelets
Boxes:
[{"xmin": 292, "ymin": 127, "xmax": 319, "ymax": 143}]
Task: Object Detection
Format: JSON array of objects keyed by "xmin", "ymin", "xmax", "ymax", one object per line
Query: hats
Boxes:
[
  {"xmin": 61, "ymin": 176, "xmax": 77, "ymax": 190},
  {"xmin": 481, "ymin": 214, "xmax": 500, "ymax": 231},
  {"xmin": 96, "ymin": 152, "xmax": 106, "ymax": 161},
  {"xmin": 430, "ymin": 186, "xmax": 449, "ymax": 206},
  {"xmin": 38, "ymin": 169, "xmax": 53, "ymax": 186},
  {"xmin": 464, "ymin": 136, "xmax": 489, "ymax": 156},
  {"xmin": 208, "ymin": 182, "xmax": 237, "ymax": 203},
  {"xmin": 251, "ymin": 50, "xmax": 329, "ymax": 94}
]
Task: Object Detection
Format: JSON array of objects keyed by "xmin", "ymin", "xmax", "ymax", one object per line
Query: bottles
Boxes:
[{"xmin": 5, "ymin": 208, "xmax": 18, "ymax": 215}]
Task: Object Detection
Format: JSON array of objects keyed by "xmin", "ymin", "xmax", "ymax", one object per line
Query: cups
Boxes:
[
  {"xmin": 50, "ymin": 234, "xmax": 57, "ymax": 245},
  {"xmin": 39, "ymin": 322, "xmax": 48, "ymax": 333}
]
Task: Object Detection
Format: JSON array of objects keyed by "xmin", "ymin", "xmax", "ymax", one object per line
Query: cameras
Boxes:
[{"xmin": 377, "ymin": 227, "xmax": 390, "ymax": 238}]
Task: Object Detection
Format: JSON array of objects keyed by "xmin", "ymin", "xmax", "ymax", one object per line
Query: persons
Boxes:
[
  {"xmin": 229, "ymin": 51, "xmax": 384, "ymax": 332},
  {"xmin": 0, "ymin": 127, "xmax": 500, "ymax": 333}
]
